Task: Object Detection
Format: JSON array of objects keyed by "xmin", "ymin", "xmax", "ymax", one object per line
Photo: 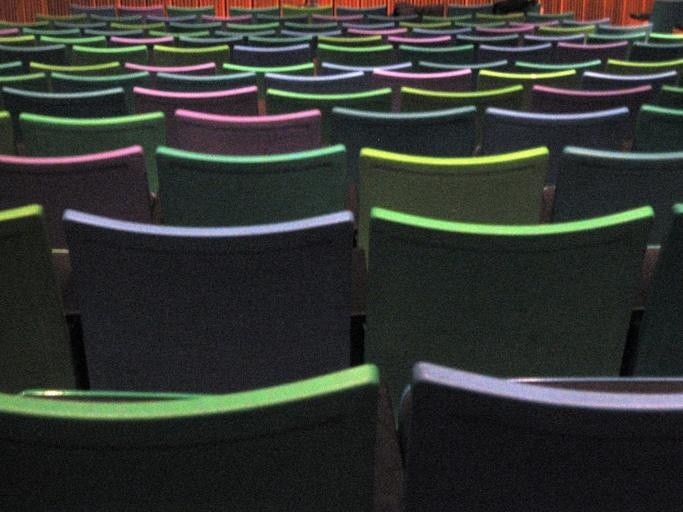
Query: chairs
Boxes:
[{"xmin": 1, "ymin": 0, "xmax": 682, "ymax": 512}]
